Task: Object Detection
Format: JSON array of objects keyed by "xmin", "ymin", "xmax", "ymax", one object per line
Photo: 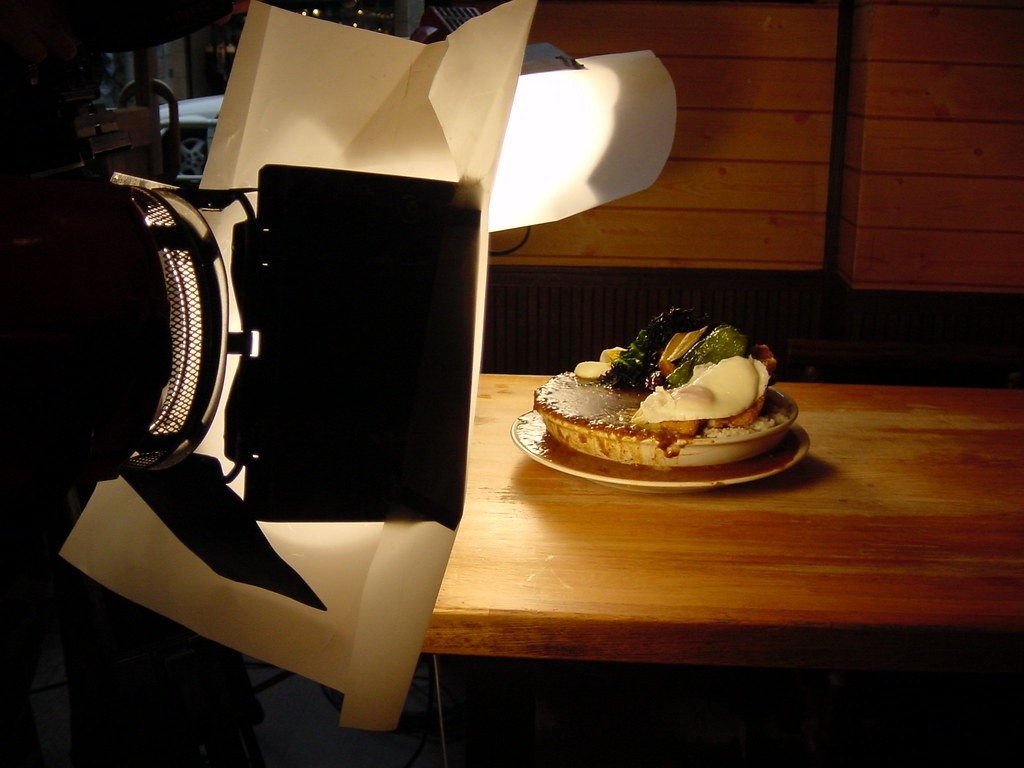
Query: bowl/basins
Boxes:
[{"xmin": 541, "ymin": 392, "xmax": 797, "ymax": 466}]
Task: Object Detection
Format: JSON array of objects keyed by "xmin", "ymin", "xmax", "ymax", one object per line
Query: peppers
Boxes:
[{"xmin": 664, "ymin": 324, "xmax": 748, "ymax": 389}]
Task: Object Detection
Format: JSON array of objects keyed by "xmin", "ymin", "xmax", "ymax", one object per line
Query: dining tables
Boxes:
[{"xmin": 418, "ymin": 373, "xmax": 1024, "ymax": 768}]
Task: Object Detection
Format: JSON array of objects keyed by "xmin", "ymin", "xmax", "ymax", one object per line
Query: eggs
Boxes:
[
  {"xmin": 599, "ymin": 346, "xmax": 628, "ymax": 363},
  {"xmin": 640, "ymin": 355, "xmax": 769, "ymax": 423}
]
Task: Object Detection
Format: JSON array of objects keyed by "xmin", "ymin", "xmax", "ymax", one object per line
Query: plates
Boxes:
[{"xmin": 510, "ymin": 410, "xmax": 809, "ymax": 492}]
